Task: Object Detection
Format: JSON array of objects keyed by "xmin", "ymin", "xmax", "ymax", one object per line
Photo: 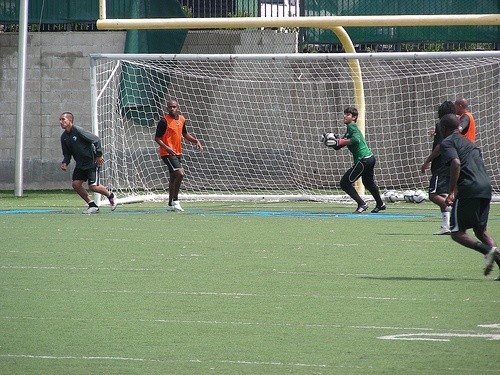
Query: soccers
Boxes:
[
  {"xmin": 322, "ymin": 133, "xmax": 337, "ymax": 146},
  {"xmin": 404, "ymin": 190, "xmax": 415, "ymax": 203},
  {"xmin": 413, "ymin": 190, "xmax": 427, "ymax": 203},
  {"xmin": 384, "ymin": 192, "xmax": 397, "ymax": 202}
]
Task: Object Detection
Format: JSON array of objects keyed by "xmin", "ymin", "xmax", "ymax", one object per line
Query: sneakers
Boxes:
[
  {"xmin": 352, "ymin": 203, "xmax": 368, "ymax": 214},
  {"xmin": 171, "ymin": 199, "xmax": 184, "ymax": 212},
  {"xmin": 82, "ymin": 207, "xmax": 99, "ymax": 214},
  {"xmin": 494, "ymin": 274, "xmax": 500, "ymax": 280},
  {"xmin": 433, "ymin": 227, "xmax": 451, "ymax": 235},
  {"xmin": 167, "ymin": 205, "xmax": 175, "ymax": 211},
  {"xmin": 371, "ymin": 203, "xmax": 386, "ymax": 213},
  {"xmin": 483, "ymin": 247, "xmax": 498, "ymax": 276},
  {"xmin": 109, "ymin": 192, "xmax": 117, "ymax": 210}
]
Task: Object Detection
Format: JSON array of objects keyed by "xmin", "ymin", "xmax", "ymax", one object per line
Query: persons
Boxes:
[
  {"xmin": 421, "ymin": 98, "xmax": 500, "ymax": 280},
  {"xmin": 322, "ymin": 108, "xmax": 386, "ymax": 213},
  {"xmin": 155, "ymin": 100, "xmax": 202, "ymax": 211},
  {"xmin": 59, "ymin": 111, "xmax": 117, "ymax": 214}
]
